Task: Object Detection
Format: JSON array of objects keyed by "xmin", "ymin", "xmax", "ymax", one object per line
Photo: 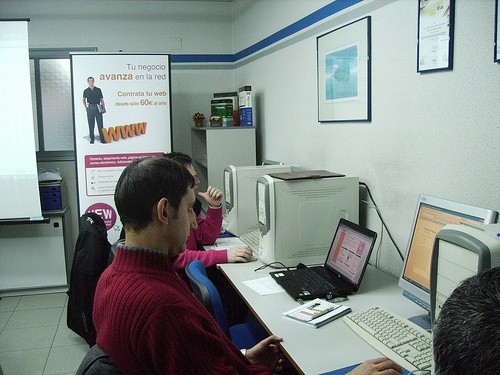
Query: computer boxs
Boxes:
[
  {"xmin": 254, "ymin": 174, "xmax": 360, "ymax": 268},
  {"xmin": 431, "ymin": 223, "xmax": 500, "ymax": 331},
  {"xmin": 221, "ymin": 165, "xmax": 291, "ymax": 237}
]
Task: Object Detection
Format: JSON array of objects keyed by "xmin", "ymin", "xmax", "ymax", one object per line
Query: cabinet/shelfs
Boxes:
[{"xmin": 190, "ymin": 125, "xmax": 257, "ymax": 218}]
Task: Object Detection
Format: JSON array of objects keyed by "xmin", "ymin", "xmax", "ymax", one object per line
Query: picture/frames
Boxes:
[{"xmin": 316, "ymin": 15, "xmax": 372, "ymax": 123}]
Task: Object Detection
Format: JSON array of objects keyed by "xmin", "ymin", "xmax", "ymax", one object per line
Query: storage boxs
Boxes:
[{"xmin": 38, "ymin": 180, "xmax": 69, "ymax": 211}]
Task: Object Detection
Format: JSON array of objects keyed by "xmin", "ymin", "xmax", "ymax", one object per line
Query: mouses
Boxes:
[{"xmin": 240, "ymin": 255, "xmax": 257, "ymax": 262}]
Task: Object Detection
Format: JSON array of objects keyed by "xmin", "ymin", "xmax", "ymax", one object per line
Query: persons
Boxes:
[
  {"xmin": 83, "ymin": 77, "xmax": 107, "ymax": 144},
  {"xmin": 434, "ymin": 267, "xmax": 500, "ymax": 375},
  {"xmin": 163, "ymin": 152, "xmax": 252, "ymax": 270},
  {"xmin": 94, "ymin": 156, "xmax": 403, "ymax": 375}
]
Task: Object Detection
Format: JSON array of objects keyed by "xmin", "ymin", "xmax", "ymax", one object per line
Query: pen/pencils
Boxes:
[{"xmin": 311, "ymin": 307, "xmax": 334, "ymax": 319}]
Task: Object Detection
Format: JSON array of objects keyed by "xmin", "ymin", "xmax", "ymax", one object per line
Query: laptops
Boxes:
[{"xmin": 269, "ymin": 218, "xmax": 377, "ymax": 301}]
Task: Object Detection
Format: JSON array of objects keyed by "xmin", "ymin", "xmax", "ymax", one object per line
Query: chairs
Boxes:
[{"xmin": 185, "ymin": 260, "xmax": 269, "ymax": 350}]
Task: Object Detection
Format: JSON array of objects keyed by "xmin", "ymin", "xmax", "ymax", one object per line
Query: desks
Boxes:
[
  {"xmin": 0, "ymin": 205, "xmax": 70, "ymax": 298},
  {"xmin": 219, "ymin": 260, "xmax": 432, "ymax": 375},
  {"xmin": 203, "ymin": 237, "xmax": 257, "ymax": 270}
]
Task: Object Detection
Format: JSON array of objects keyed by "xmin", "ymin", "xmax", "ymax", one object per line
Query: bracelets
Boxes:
[{"xmin": 241, "ymin": 349, "xmax": 246, "ymax": 355}]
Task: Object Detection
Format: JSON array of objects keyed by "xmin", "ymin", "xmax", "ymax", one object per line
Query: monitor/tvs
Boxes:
[{"xmin": 398, "ymin": 195, "xmax": 497, "ymax": 332}]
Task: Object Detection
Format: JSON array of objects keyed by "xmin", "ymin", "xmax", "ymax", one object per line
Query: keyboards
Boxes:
[
  {"xmin": 345, "ymin": 306, "xmax": 435, "ymax": 375},
  {"xmin": 213, "ymin": 237, "xmax": 248, "ymax": 250}
]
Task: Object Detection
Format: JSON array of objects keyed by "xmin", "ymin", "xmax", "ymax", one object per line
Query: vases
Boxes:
[
  {"xmin": 195, "ymin": 120, "xmax": 203, "ymax": 127},
  {"xmin": 192, "ymin": 113, "xmax": 205, "ymax": 122}
]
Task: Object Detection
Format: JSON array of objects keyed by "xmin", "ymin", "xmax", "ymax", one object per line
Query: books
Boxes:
[
  {"xmin": 283, "ymin": 298, "xmax": 351, "ymax": 329},
  {"xmin": 97, "ymin": 104, "xmax": 103, "ymax": 112}
]
